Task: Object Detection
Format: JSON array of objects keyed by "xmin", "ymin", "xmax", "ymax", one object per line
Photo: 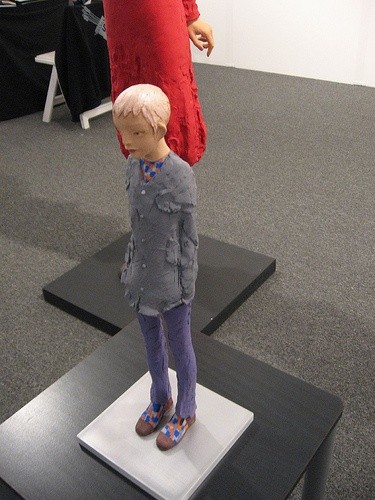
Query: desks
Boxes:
[{"xmin": 0, "ymin": 0, "xmax": 67, "ymax": 121}]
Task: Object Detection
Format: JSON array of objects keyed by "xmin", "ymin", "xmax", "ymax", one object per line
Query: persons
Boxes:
[
  {"xmin": 111, "ymin": 83, "xmax": 200, "ymax": 452},
  {"xmin": 102, "ymin": 0, "xmax": 215, "ymax": 167}
]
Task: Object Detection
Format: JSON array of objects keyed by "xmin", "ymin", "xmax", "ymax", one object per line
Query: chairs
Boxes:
[{"xmin": 34, "ymin": 50, "xmax": 112, "ymax": 130}]
